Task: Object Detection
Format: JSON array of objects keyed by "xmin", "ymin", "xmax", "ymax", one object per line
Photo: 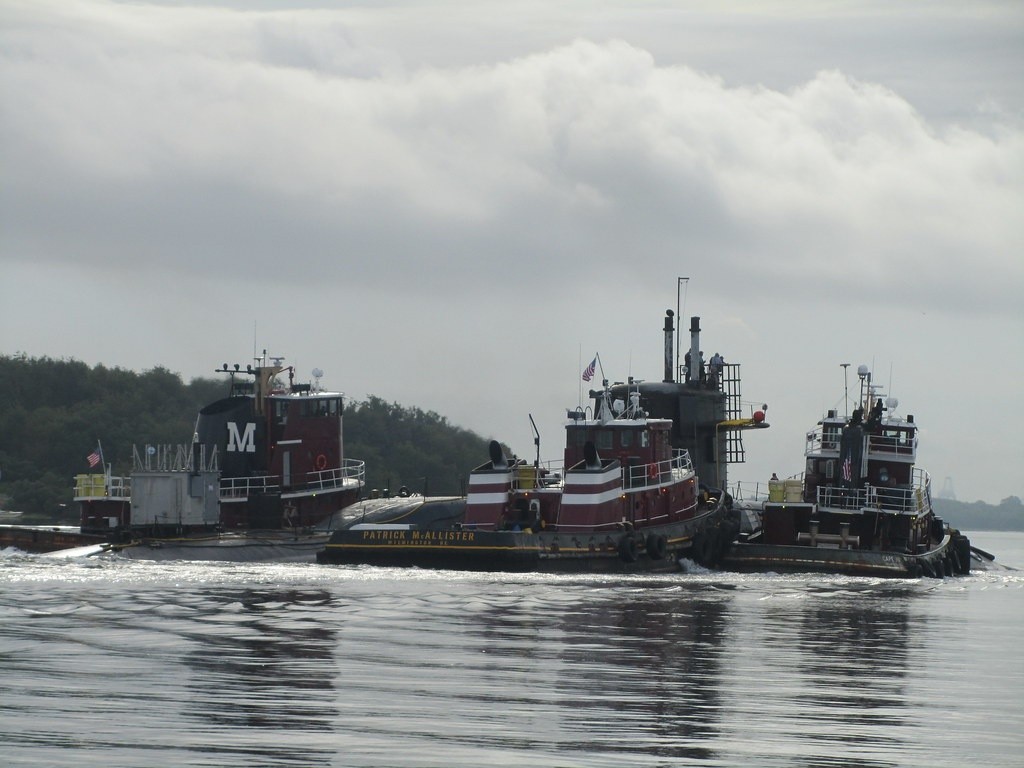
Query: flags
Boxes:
[
  {"xmin": 87, "ymin": 447, "xmax": 102, "ymax": 467},
  {"xmin": 582, "ymin": 358, "xmax": 596, "ymax": 381},
  {"xmin": 842, "ymin": 448, "xmax": 853, "ymax": 483}
]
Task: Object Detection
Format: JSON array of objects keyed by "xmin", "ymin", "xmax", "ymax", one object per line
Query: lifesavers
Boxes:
[
  {"xmin": 649, "ymin": 463, "xmax": 658, "ymax": 480},
  {"xmin": 317, "ymin": 455, "xmax": 326, "ymax": 469}
]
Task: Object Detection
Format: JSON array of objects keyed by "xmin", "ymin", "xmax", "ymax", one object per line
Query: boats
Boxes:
[{"xmin": 1, "ymin": 279, "xmax": 1008, "ymax": 578}]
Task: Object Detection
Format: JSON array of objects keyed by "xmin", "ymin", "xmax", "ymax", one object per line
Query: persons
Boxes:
[
  {"xmin": 709, "ymin": 353, "xmax": 724, "ymax": 388},
  {"xmin": 282, "ymin": 403, "xmax": 289, "ymax": 415},
  {"xmin": 884, "ymin": 476, "xmax": 908, "ymax": 509},
  {"xmin": 684, "ymin": 348, "xmax": 692, "ymax": 383},
  {"xmin": 697, "ymin": 350, "xmax": 706, "ymax": 380}
]
